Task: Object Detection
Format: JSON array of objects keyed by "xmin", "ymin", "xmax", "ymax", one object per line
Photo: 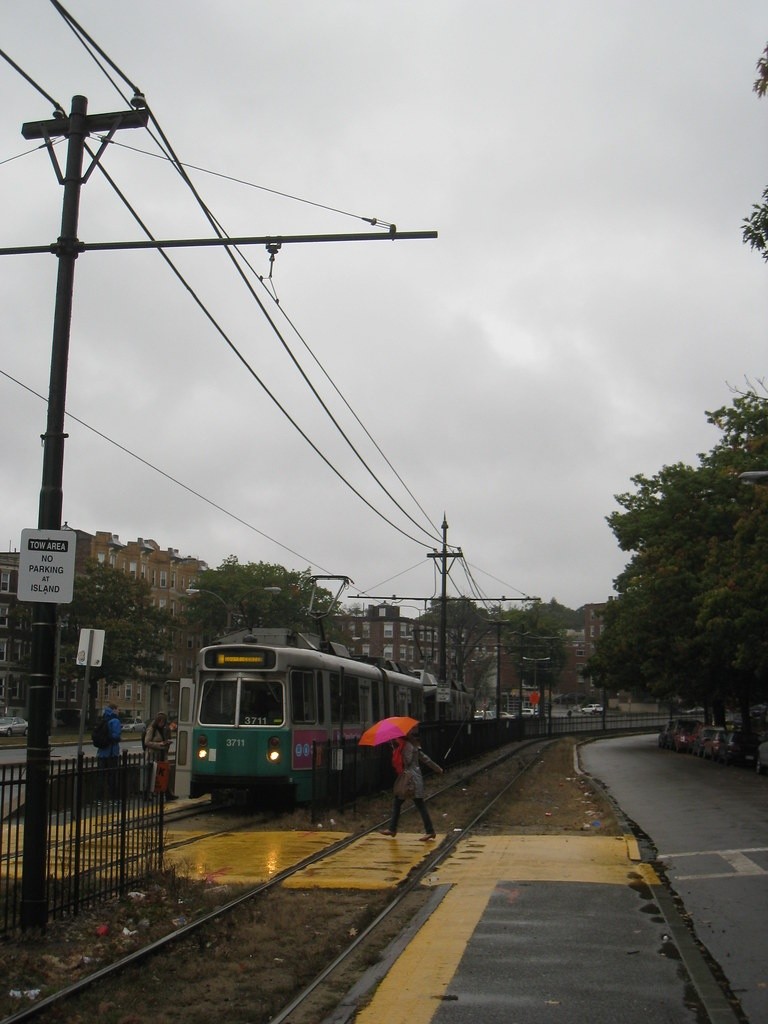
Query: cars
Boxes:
[
  {"xmin": 682, "ymin": 706, "xmax": 705, "ymax": 716},
  {"xmin": 755, "ymin": 739, "xmax": 768, "ymax": 776},
  {"xmin": 0, "ymin": 716, "xmax": 28, "ymax": 738},
  {"xmin": 121, "ymin": 717, "xmax": 147, "ymax": 733},
  {"xmin": 657, "ymin": 718, "xmax": 764, "ymax": 767},
  {"xmin": 474, "ymin": 706, "xmax": 539, "ymax": 720}
]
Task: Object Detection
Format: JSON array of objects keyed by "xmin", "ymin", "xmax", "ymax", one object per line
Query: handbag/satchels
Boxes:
[{"xmin": 393, "ymin": 771, "xmax": 416, "ymax": 799}]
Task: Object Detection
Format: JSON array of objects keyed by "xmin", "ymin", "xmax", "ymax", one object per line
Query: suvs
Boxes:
[{"xmin": 581, "ymin": 704, "xmax": 604, "ymax": 715}]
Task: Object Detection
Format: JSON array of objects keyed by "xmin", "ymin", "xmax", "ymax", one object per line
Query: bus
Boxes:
[{"xmin": 172, "ymin": 574, "xmax": 475, "ymax": 806}]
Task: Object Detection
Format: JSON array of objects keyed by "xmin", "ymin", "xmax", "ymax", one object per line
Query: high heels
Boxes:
[
  {"xmin": 379, "ymin": 830, "xmax": 396, "ymax": 837},
  {"xmin": 419, "ymin": 833, "xmax": 437, "ymax": 841}
]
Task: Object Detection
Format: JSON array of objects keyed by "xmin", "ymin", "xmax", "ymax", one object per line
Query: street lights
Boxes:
[
  {"xmin": 184, "ymin": 586, "xmax": 282, "ymax": 634},
  {"xmin": 351, "ymin": 634, "xmax": 415, "ymax": 657},
  {"xmin": 523, "ymin": 656, "xmax": 551, "ymax": 719}
]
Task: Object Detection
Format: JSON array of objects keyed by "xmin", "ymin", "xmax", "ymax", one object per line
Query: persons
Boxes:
[
  {"xmin": 380, "ymin": 729, "xmax": 444, "ymax": 842},
  {"xmin": 143, "ymin": 712, "xmax": 179, "ymax": 802},
  {"xmin": 93, "ymin": 703, "xmax": 124, "ymax": 807}
]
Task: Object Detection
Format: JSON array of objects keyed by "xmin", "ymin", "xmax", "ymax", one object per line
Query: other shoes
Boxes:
[
  {"xmin": 108, "ymin": 800, "xmax": 122, "ymax": 805},
  {"xmin": 94, "ymin": 801, "xmax": 105, "ymax": 808}
]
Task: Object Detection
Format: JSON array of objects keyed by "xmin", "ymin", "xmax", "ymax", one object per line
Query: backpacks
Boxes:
[
  {"xmin": 141, "ymin": 725, "xmax": 156, "ymax": 751},
  {"xmin": 91, "ymin": 714, "xmax": 120, "ymax": 748}
]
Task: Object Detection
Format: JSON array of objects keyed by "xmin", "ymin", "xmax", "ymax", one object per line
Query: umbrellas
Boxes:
[{"xmin": 359, "ymin": 716, "xmax": 421, "ymax": 748}]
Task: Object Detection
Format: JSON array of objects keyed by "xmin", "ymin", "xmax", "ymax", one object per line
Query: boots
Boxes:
[{"xmin": 165, "ymin": 787, "xmax": 179, "ymax": 801}]
